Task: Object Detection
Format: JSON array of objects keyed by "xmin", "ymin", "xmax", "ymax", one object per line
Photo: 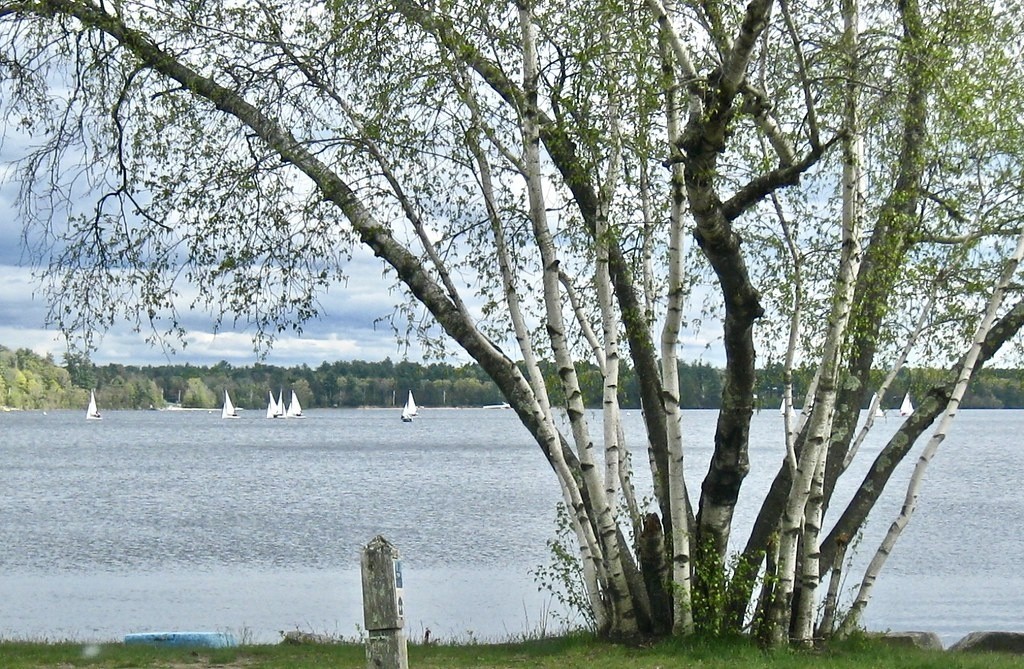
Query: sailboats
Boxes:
[
  {"xmin": 221, "ymin": 389, "xmax": 241, "ymax": 419},
  {"xmin": 868, "ymin": 392, "xmax": 885, "ymax": 417},
  {"xmin": 401, "ymin": 389, "xmax": 420, "ymax": 422},
  {"xmin": 899, "ymin": 392, "xmax": 914, "ymax": 417},
  {"xmin": 86, "ymin": 391, "xmax": 102, "ymax": 420},
  {"xmin": 266, "ymin": 389, "xmax": 306, "ymax": 420}
]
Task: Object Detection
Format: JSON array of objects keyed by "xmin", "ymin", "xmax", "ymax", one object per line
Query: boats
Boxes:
[{"xmin": 483, "ymin": 404, "xmax": 511, "ymax": 409}]
